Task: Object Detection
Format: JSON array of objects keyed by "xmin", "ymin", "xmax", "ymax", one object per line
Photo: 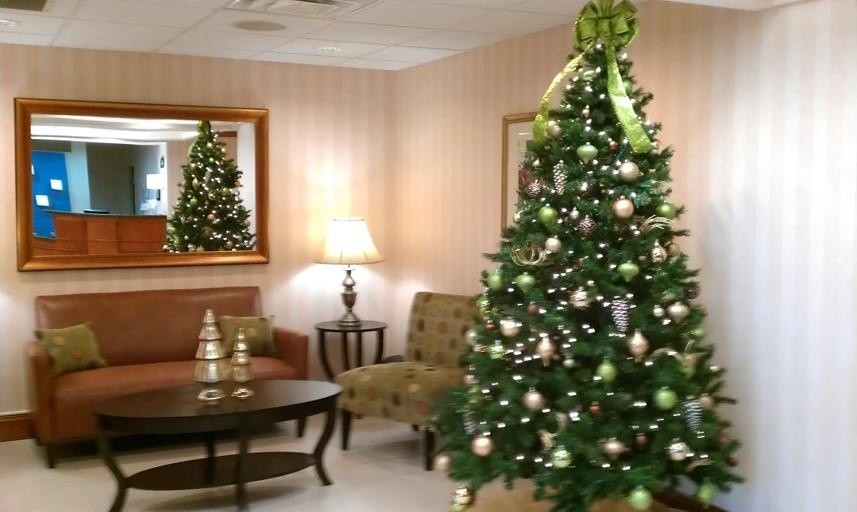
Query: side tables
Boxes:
[{"xmin": 314, "ymin": 321, "xmax": 388, "ymax": 451}]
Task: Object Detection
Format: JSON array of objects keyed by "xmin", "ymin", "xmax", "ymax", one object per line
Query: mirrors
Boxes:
[{"xmin": 12, "ymin": 96, "xmax": 268, "ymax": 272}]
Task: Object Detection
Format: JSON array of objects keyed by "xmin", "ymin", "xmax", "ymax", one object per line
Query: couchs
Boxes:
[{"xmin": 21, "ymin": 285, "xmax": 313, "ymax": 468}]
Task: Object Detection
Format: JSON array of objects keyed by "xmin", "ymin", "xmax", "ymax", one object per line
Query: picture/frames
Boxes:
[{"xmin": 500, "ymin": 111, "xmax": 542, "ymax": 234}]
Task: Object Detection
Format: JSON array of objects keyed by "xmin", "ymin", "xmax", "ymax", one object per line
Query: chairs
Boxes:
[{"xmin": 332, "ymin": 290, "xmax": 491, "ymax": 472}]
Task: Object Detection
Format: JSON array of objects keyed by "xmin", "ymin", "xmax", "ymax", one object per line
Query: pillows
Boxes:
[
  {"xmin": 33, "ymin": 316, "xmax": 110, "ymax": 380},
  {"xmin": 214, "ymin": 313, "xmax": 281, "ymax": 359}
]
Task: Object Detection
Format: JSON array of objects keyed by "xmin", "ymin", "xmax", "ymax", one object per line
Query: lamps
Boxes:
[{"xmin": 316, "ymin": 216, "xmax": 386, "ymax": 328}]
